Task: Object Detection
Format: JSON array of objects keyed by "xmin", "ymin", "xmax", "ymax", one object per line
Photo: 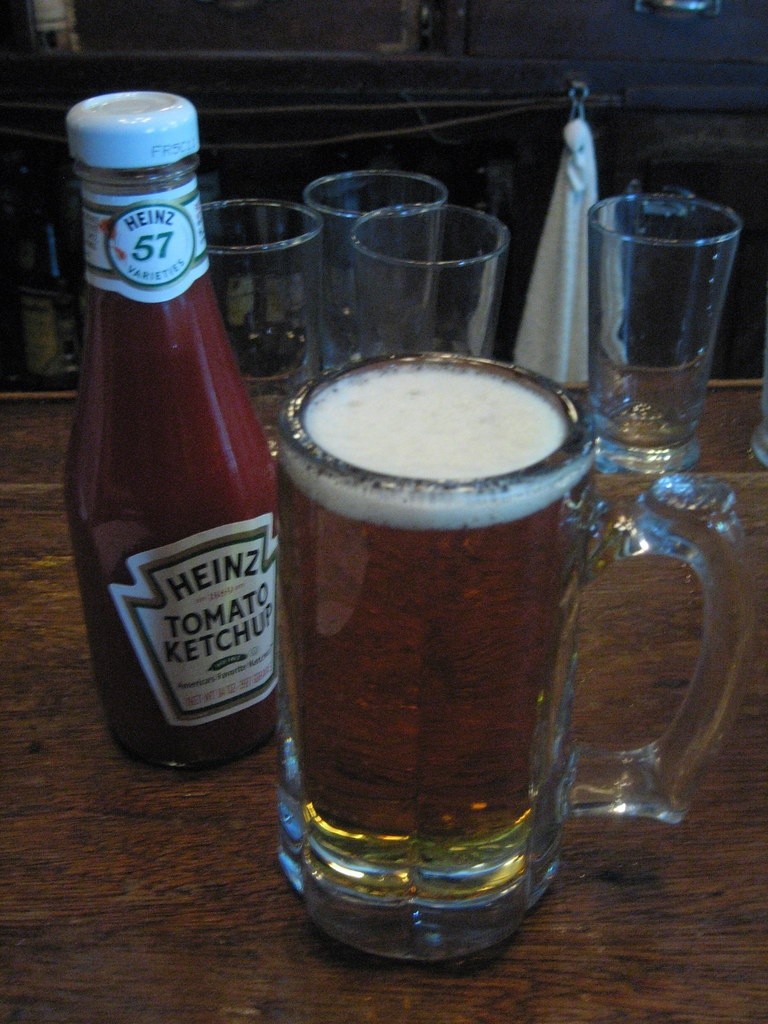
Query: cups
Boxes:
[
  {"xmin": 265, "ymin": 349, "xmax": 753, "ymax": 965},
  {"xmin": 582, "ymin": 201, "xmax": 742, "ymax": 475},
  {"xmin": 350, "ymin": 203, "xmax": 513, "ymax": 362},
  {"xmin": 300, "ymin": 169, "xmax": 448, "ymax": 367},
  {"xmin": 198, "ymin": 199, "xmax": 319, "ymax": 487}
]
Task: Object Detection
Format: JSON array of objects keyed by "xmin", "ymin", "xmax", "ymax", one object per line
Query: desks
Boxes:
[{"xmin": 0, "ymin": 380, "xmax": 766, "ymax": 1024}]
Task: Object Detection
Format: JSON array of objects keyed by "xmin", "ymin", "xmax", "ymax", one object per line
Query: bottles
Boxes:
[{"xmin": 64, "ymin": 90, "xmax": 283, "ymax": 772}]
